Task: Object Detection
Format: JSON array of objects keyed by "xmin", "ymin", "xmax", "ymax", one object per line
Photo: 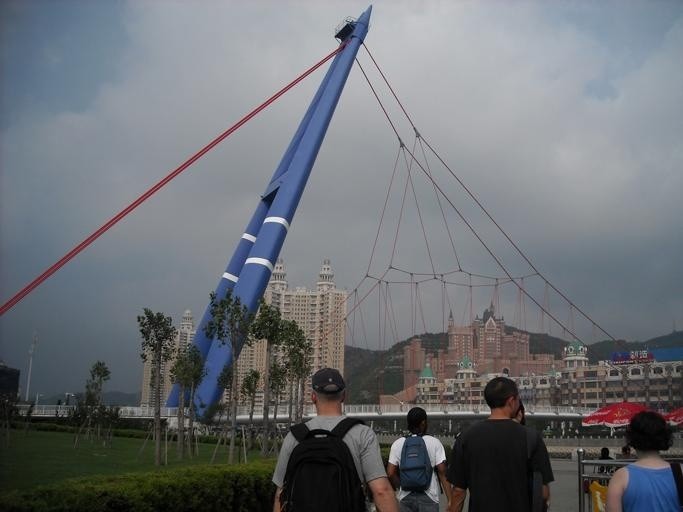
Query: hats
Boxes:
[{"xmin": 312, "ymin": 368, "xmax": 345, "ymax": 394}]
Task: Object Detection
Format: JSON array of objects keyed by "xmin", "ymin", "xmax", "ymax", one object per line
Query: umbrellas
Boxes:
[
  {"xmin": 662, "ymin": 406, "xmax": 683, "ymax": 426},
  {"xmin": 580, "ymin": 401, "xmax": 653, "ymax": 427}
]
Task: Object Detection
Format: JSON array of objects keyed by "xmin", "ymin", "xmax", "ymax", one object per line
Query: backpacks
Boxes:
[
  {"xmin": 279, "ymin": 417, "xmax": 365, "ymax": 512},
  {"xmin": 399, "ymin": 434, "xmax": 433, "ymax": 490}
]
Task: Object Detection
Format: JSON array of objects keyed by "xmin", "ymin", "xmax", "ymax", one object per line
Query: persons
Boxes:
[
  {"xmin": 511, "ymin": 398, "xmax": 526, "ymax": 426},
  {"xmin": 606, "ymin": 410, "xmax": 683, "ymax": 511},
  {"xmin": 598, "ymin": 446, "xmax": 613, "ymax": 459},
  {"xmin": 616, "ymin": 446, "xmax": 636, "ymax": 458},
  {"xmin": 443, "ymin": 377, "xmax": 554, "ymax": 511},
  {"xmin": 386, "ymin": 406, "xmax": 452, "ymax": 511},
  {"xmin": 269, "ymin": 367, "xmax": 400, "ymax": 511}
]
